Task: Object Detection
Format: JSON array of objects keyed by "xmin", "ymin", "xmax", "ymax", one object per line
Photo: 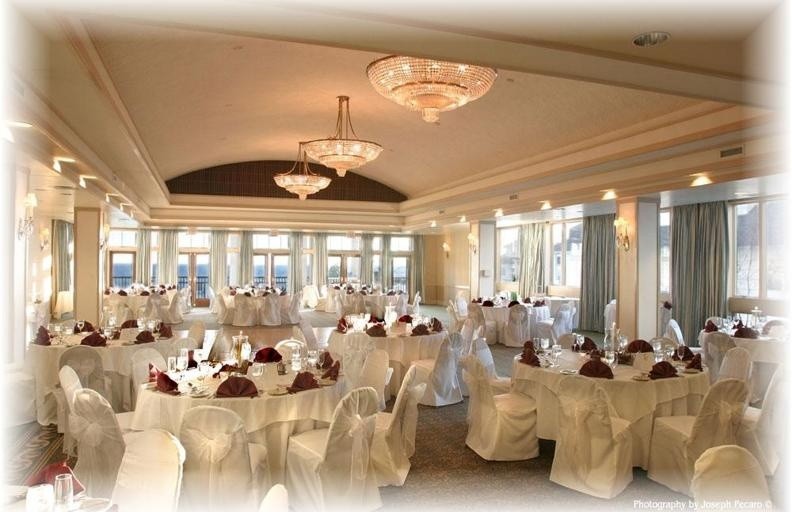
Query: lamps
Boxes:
[
  {"xmin": 18, "ymin": 194, "xmax": 50, "ymax": 251},
  {"xmin": 613, "ymin": 217, "xmax": 631, "ymax": 251},
  {"xmin": 272, "ymin": 54, "xmax": 499, "ymax": 202}
]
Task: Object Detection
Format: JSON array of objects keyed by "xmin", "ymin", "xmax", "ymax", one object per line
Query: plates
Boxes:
[
  {"xmin": 188, "ymin": 392, "xmax": 210, "ymax": 398},
  {"xmin": 633, "ymin": 376, "xmax": 650, "ymax": 381},
  {"xmin": 267, "ymin": 390, "xmax": 289, "ymax": 396},
  {"xmin": 686, "ymin": 369, "xmax": 699, "ymax": 373}
]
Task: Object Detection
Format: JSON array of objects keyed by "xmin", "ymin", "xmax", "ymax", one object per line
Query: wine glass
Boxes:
[
  {"xmin": 714, "ymin": 308, "xmax": 764, "ymax": 333},
  {"xmin": 533, "ymin": 329, "xmax": 685, "ymax": 376},
  {"xmin": 45, "ymin": 313, "xmax": 161, "ymax": 345},
  {"xmin": 166, "ymin": 335, "xmax": 325, "ymax": 390}
]
